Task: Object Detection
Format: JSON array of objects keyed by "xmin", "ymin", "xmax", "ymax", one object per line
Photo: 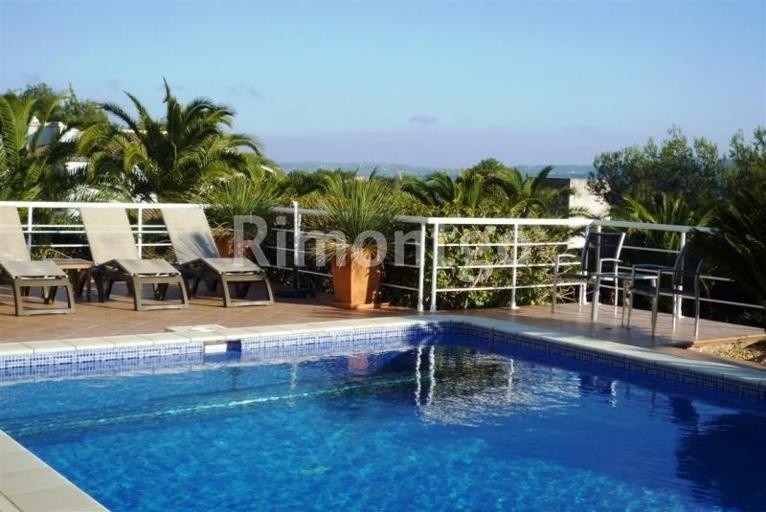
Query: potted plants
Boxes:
[
  {"xmin": 182, "ymin": 168, "xmax": 297, "ymax": 260},
  {"xmin": 301, "ymin": 183, "xmax": 406, "ymax": 312}
]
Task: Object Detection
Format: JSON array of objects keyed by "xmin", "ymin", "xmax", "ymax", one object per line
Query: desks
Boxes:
[
  {"xmin": 48, "ymin": 258, "xmax": 91, "ymax": 303},
  {"xmin": 589, "ymin": 271, "xmax": 659, "ymax": 331}
]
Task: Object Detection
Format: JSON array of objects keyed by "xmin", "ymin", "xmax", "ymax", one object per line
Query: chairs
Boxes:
[
  {"xmin": 0, "ymin": 206, "xmax": 77, "ymax": 316},
  {"xmin": 160, "ymin": 205, "xmax": 276, "ymax": 307},
  {"xmin": 548, "ymin": 229, "xmax": 627, "ymax": 322},
  {"xmin": 628, "ymin": 242, "xmax": 708, "ymax": 338},
  {"xmin": 76, "ymin": 205, "xmax": 192, "ymax": 311}
]
